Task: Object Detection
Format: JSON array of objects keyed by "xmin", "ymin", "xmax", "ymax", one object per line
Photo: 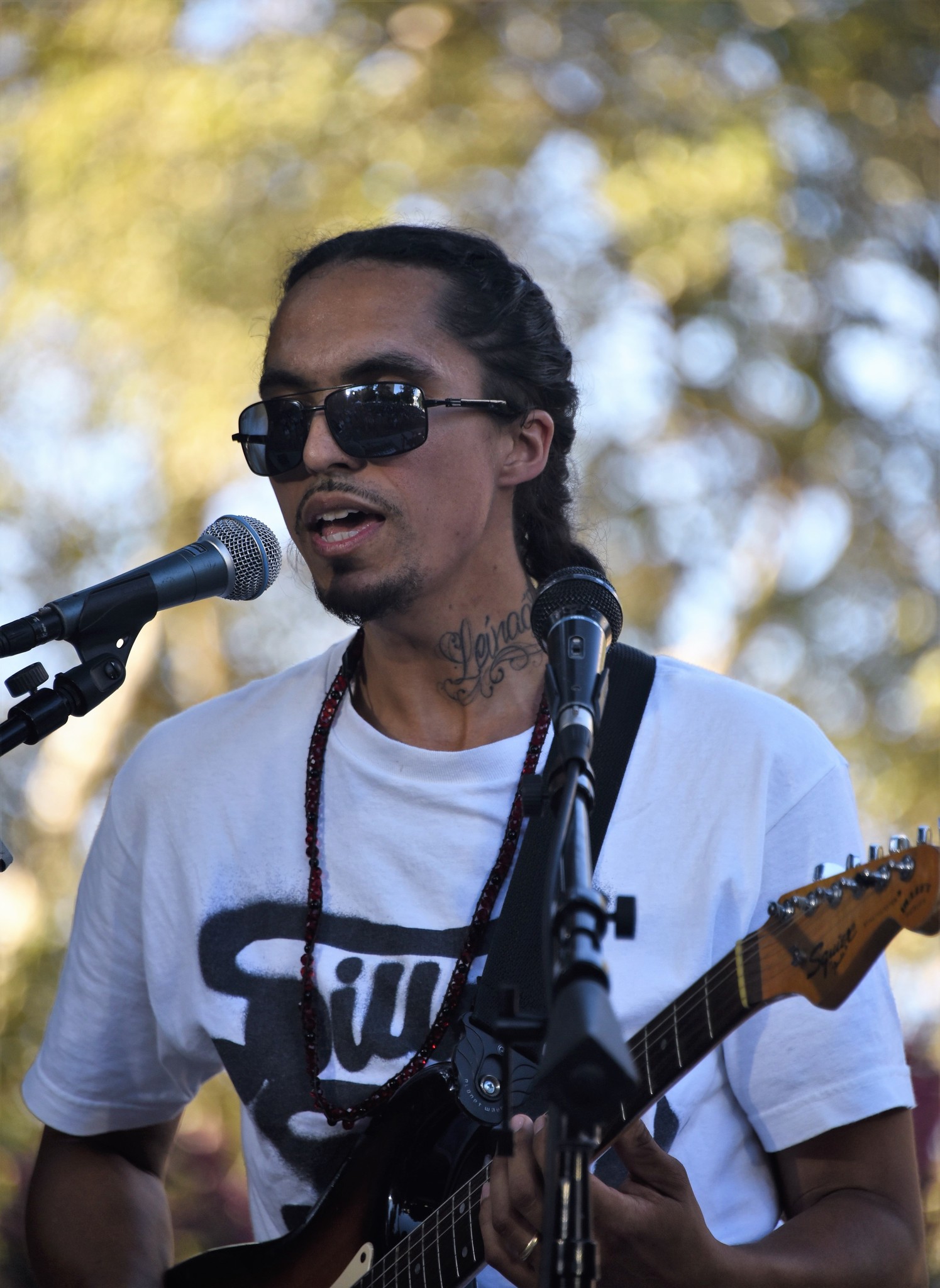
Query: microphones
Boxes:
[
  {"xmin": 0, "ymin": 513, "xmax": 283, "ymax": 658},
  {"xmin": 529, "ymin": 567, "xmax": 623, "ymax": 775}
]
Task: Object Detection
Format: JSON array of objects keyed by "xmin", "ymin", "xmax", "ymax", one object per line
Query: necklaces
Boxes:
[{"xmin": 300, "ymin": 636, "xmax": 561, "ymax": 1132}]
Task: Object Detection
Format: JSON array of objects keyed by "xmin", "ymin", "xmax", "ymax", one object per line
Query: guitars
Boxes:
[{"xmin": 163, "ymin": 821, "xmax": 939, "ymax": 1288}]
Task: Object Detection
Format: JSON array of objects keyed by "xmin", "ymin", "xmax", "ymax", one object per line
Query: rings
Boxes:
[{"xmin": 518, "ymin": 1227, "xmax": 541, "ymax": 1268}]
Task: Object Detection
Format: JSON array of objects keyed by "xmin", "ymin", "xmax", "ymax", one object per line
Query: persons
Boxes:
[{"xmin": 21, "ymin": 223, "xmax": 929, "ymax": 1288}]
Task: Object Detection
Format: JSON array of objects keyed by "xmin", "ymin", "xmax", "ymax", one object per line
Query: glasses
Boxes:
[{"xmin": 228, "ymin": 381, "xmax": 507, "ymax": 477}]
